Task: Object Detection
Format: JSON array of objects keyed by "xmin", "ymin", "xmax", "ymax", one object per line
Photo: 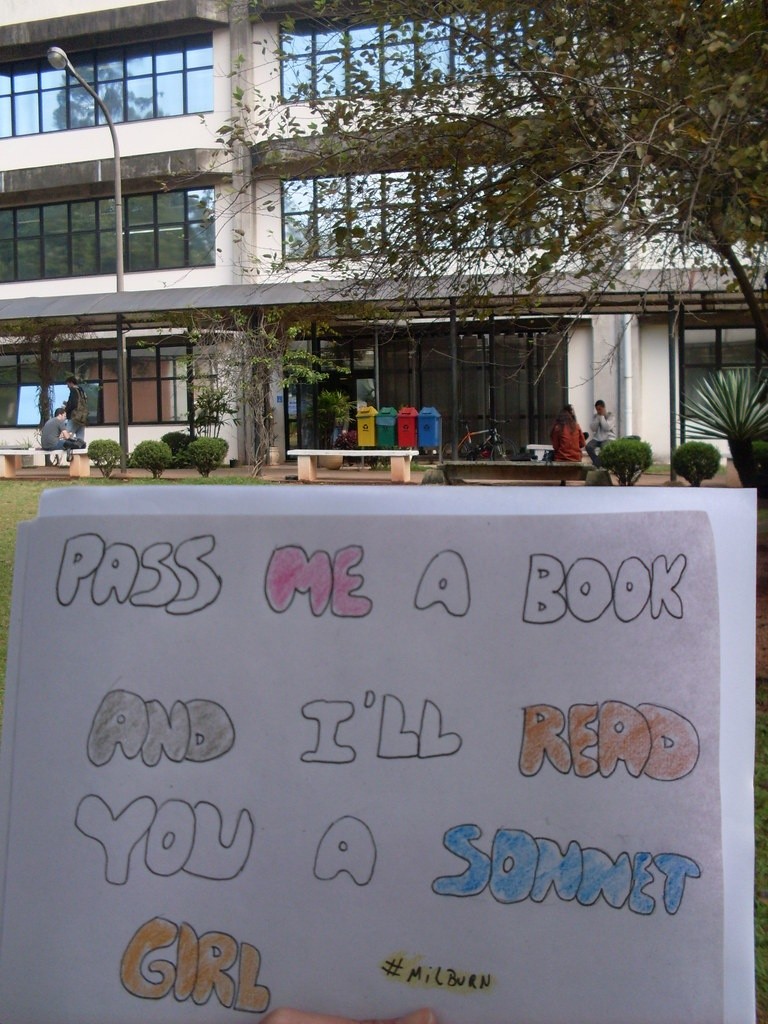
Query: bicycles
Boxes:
[{"xmin": 441, "ymin": 414, "xmax": 520, "ymax": 462}]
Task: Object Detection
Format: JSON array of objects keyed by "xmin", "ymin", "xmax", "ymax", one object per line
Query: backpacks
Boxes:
[{"xmin": 63, "ymin": 436, "xmax": 86, "ymax": 462}]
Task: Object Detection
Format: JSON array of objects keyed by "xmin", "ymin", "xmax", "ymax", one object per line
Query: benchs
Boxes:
[
  {"xmin": 438, "ymin": 460, "xmax": 596, "ymax": 485},
  {"xmin": 287, "ymin": 449, "xmax": 418, "ymax": 482},
  {"xmin": 0, "ymin": 449, "xmax": 91, "ymax": 477}
]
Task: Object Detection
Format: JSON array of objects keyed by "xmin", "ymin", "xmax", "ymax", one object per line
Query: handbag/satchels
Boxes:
[
  {"xmin": 542, "ymin": 450, "xmax": 553, "ymax": 461},
  {"xmin": 70, "ymin": 388, "xmax": 88, "ymax": 426}
]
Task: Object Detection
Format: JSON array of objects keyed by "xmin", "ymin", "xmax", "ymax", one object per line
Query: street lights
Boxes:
[{"xmin": 47, "ymin": 45, "xmax": 129, "ymax": 474}]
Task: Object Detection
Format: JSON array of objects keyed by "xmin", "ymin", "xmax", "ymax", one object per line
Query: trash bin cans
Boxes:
[
  {"xmin": 374, "ymin": 407, "xmax": 398, "ymax": 446},
  {"xmin": 355, "ymin": 406, "xmax": 378, "ymax": 446},
  {"xmin": 418, "ymin": 407, "xmax": 441, "ymax": 446},
  {"xmin": 396, "ymin": 407, "xmax": 419, "ymax": 446}
]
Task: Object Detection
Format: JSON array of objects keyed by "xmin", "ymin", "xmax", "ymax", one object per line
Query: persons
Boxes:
[
  {"xmin": 42, "ymin": 408, "xmax": 70, "ymax": 451},
  {"xmin": 586, "ymin": 400, "xmax": 617, "ymax": 470},
  {"xmin": 55, "ymin": 377, "xmax": 88, "ymax": 465},
  {"xmin": 548, "ymin": 401, "xmax": 585, "ymax": 485}
]
